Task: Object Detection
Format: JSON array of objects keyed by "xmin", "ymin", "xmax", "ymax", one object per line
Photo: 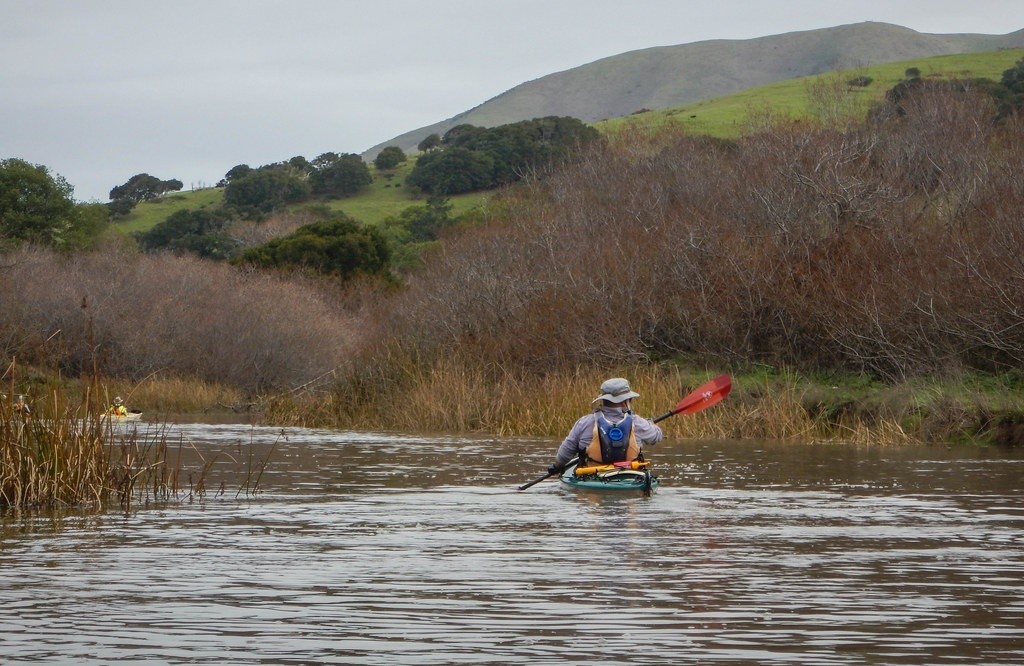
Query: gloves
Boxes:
[{"xmin": 548, "ymin": 464, "xmax": 565, "ymax": 477}]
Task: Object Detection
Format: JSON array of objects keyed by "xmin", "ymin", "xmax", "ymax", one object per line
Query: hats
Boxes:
[{"xmin": 591, "ymin": 378, "xmax": 640, "ymax": 403}]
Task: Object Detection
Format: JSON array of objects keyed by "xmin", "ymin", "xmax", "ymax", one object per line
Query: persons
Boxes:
[
  {"xmin": 12, "ymin": 396, "xmax": 30, "ymax": 414},
  {"xmin": 105, "ymin": 396, "xmax": 127, "ymax": 417},
  {"xmin": 555, "ymin": 377, "xmax": 662, "ymax": 470}
]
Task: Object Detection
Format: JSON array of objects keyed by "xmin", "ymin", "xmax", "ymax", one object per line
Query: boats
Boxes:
[
  {"xmin": 558, "ymin": 456, "xmax": 659, "ymax": 492},
  {"xmin": 99, "ymin": 408, "xmax": 143, "ymax": 423}
]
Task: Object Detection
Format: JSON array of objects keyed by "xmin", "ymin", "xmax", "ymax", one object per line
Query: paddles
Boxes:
[{"xmin": 518, "ymin": 373, "xmax": 732, "ymax": 495}]
